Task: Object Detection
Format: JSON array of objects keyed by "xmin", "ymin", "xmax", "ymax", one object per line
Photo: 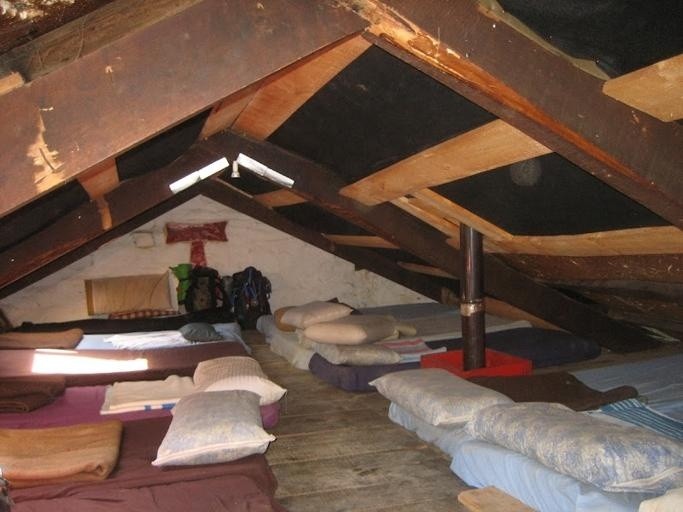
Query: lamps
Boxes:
[{"xmin": 231, "ymin": 161, "xmax": 240, "ymax": 178}]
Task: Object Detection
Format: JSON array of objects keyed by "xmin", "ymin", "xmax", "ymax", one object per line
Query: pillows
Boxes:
[
  {"xmin": 304, "ymin": 316, "xmax": 396, "ymax": 346},
  {"xmin": 368, "ymin": 368, "xmax": 515, "ymax": 427},
  {"xmin": 468, "ymin": 401, "xmax": 683, "ymax": 496},
  {"xmin": 85, "ymin": 272, "xmax": 174, "ymax": 315},
  {"xmin": 179, "ymin": 322, "xmax": 223, "ymax": 342},
  {"xmin": 151, "ymin": 390, "xmax": 276, "ymax": 470},
  {"xmin": 308, "ymin": 343, "xmax": 402, "ymax": 365},
  {"xmin": 193, "ymin": 355, "xmax": 288, "ymax": 406},
  {"xmin": 280, "ymin": 301, "xmax": 354, "ymax": 330}
]
